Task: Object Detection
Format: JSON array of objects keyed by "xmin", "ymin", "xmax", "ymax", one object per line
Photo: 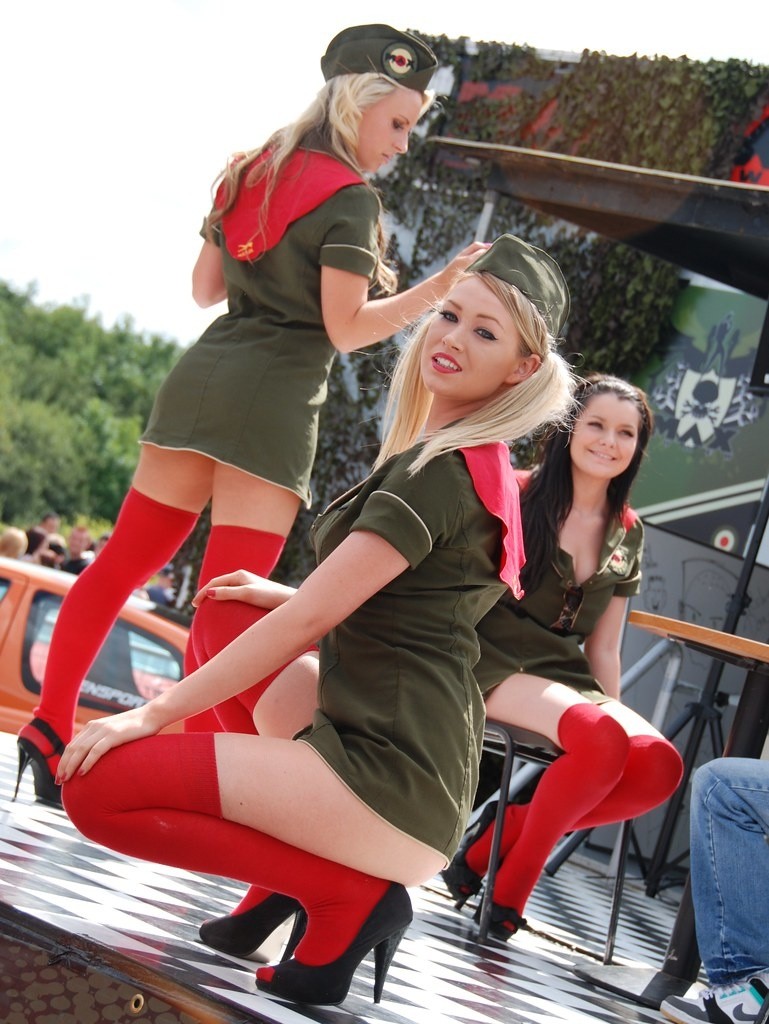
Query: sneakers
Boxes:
[{"xmin": 660, "ymin": 974, "xmax": 769, "ymax": 1024}]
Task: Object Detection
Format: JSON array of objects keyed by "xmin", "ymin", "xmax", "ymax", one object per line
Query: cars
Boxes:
[{"xmin": 0, "ymin": 554, "xmax": 193, "ymax": 739}]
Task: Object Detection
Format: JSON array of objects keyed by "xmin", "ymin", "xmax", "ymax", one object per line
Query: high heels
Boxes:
[
  {"xmin": 473, "ymin": 858, "xmax": 525, "ymax": 942},
  {"xmin": 199, "ymin": 892, "xmax": 306, "ymax": 964},
  {"xmin": 11, "ymin": 718, "xmax": 69, "ymax": 808},
  {"xmin": 255, "ymin": 881, "xmax": 413, "ymax": 1006},
  {"xmin": 441, "ymin": 801, "xmax": 516, "ymax": 910}
]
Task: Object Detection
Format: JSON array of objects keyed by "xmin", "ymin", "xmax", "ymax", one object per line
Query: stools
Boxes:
[{"xmin": 471, "ymin": 722, "xmax": 631, "ymax": 966}]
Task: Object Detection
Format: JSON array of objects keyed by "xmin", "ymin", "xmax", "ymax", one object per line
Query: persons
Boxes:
[
  {"xmin": 439, "ymin": 372, "xmax": 684, "ymax": 942},
  {"xmin": 10, "ymin": 21, "xmax": 493, "ymax": 808},
  {"xmin": 53, "ymin": 231, "xmax": 584, "ymax": 1008},
  {"xmin": 0, "ymin": 513, "xmax": 177, "ymax": 607},
  {"xmin": 660, "ymin": 755, "xmax": 769, "ymax": 1024}
]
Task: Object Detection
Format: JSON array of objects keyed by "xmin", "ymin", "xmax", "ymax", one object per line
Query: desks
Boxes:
[{"xmin": 462, "ymin": 611, "xmax": 769, "ymax": 878}]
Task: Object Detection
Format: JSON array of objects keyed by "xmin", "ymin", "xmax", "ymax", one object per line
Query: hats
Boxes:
[
  {"xmin": 463, "ymin": 233, "xmax": 573, "ymax": 342},
  {"xmin": 321, "ymin": 24, "xmax": 438, "ymax": 92}
]
